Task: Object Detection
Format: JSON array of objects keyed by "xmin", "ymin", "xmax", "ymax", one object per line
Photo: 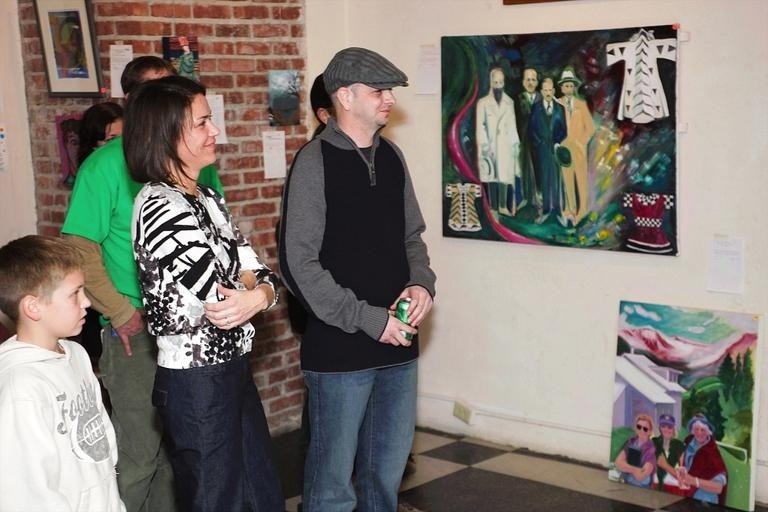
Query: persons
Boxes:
[
  {"xmin": 527, "ymin": 76, "xmax": 566, "ymax": 226},
  {"xmin": 0, "ymin": 237, "xmax": 130, "ymax": 512},
  {"xmin": 611, "ymin": 413, "xmax": 656, "ymax": 488},
  {"xmin": 74, "ymin": 99, "xmax": 128, "ymax": 170},
  {"xmin": 118, "ymin": 75, "xmax": 292, "ymax": 510},
  {"xmin": 650, "ymin": 414, "xmax": 685, "ymax": 488},
  {"xmin": 272, "ymin": 42, "xmax": 440, "ymax": 512},
  {"xmin": 515, "ymin": 68, "xmax": 539, "ymax": 213},
  {"xmin": 59, "ymin": 115, "xmax": 81, "ymax": 185},
  {"xmin": 675, "ymin": 413, "xmax": 728, "ymax": 506},
  {"xmin": 58, "ymin": 55, "xmax": 235, "ymax": 512},
  {"xmin": 471, "ymin": 65, "xmax": 519, "ymax": 222},
  {"xmin": 300, "ymin": 71, "xmax": 337, "ymax": 141},
  {"xmin": 552, "ymin": 68, "xmax": 596, "ymax": 229}
]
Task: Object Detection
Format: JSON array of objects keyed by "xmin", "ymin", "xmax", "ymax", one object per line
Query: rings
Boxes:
[{"xmin": 224, "ymin": 317, "xmax": 231, "ymax": 326}]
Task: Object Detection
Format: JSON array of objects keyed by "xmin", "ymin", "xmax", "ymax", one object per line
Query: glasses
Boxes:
[{"xmin": 636, "ymin": 423, "xmax": 651, "ymax": 432}]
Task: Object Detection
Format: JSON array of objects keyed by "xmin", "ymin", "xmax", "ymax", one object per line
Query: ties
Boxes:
[{"xmin": 530, "ymin": 94, "xmax": 573, "ymax": 117}]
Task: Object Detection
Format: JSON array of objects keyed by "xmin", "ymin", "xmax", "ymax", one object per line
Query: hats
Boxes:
[
  {"xmin": 557, "ymin": 65, "xmax": 582, "ymax": 87},
  {"xmin": 555, "ymin": 146, "xmax": 572, "ymax": 167},
  {"xmin": 687, "ymin": 412, "xmax": 716, "ymax": 434},
  {"xmin": 178, "ymin": 37, "xmax": 191, "ymax": 47},
  {"xmin": 659, "ymin": 414, "xmax": 675, "ymax": 427},
  {"xmin": 323, "ymin": 47, "xmax": 409, "ymax": 95}
]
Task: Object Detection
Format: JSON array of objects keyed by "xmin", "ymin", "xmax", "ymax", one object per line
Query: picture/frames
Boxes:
[{"xmin": 32, "ymin": 0, "xmax": 107, "ymax": 99}]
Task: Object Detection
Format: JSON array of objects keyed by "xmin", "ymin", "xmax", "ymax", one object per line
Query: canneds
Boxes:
[{"xmin": 394, "ymin": 297, "xmax": 415, "ymax": 341}]
